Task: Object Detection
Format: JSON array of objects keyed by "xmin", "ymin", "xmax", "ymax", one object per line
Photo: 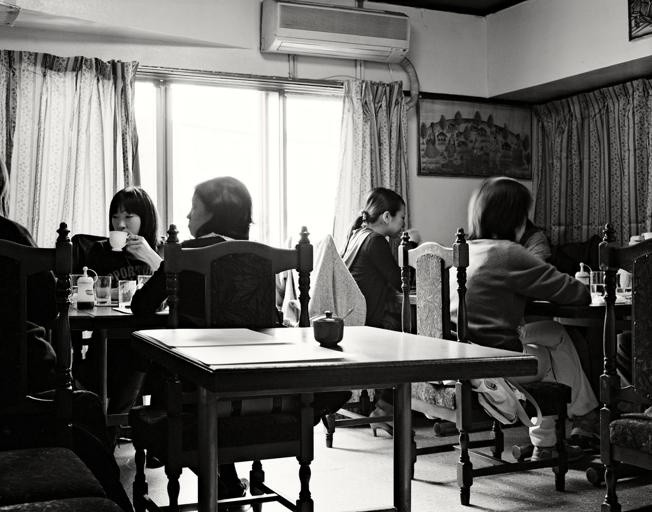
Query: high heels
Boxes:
[{"xmin": 369, "ymin": 408, "xmax": 416, "ymax": 440}]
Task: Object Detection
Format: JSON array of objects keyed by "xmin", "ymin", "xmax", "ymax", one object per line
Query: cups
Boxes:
[
  {"xmin": 94, "ymin": 276, "xmax": 111, "ymax": 305},
  {"xmin": 591, "ymin": 271, "xmax": 606, "ymax": 304},
  {"xmin": 119, "ymin": 280, "xmax": 136, "ymax": 310},
  {"xmin": 616, "ymin": 273, "xmax": 632, "ymax": 303},
  {"xmin": 109, "ymin": 231, "xmax": 132, "ymax": 252},
  {"xmin": 71, "ymin": 274, "xmax": 85, "ymax": 302}
]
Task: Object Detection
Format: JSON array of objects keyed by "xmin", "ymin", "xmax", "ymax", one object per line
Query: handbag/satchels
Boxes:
[{"xmin": 470, "ymin": 376, "xmax": 543, "ymax": 429}]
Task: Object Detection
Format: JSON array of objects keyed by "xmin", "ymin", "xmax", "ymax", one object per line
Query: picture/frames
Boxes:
[{"xmin": 415, "ymin": 100, "xmax": 534, "ymax": 180}]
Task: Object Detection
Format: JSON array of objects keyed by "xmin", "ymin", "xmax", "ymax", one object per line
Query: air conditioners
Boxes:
[{"xmin": 261, "ymin": 1, "xmax": 410, "ymax": 64}]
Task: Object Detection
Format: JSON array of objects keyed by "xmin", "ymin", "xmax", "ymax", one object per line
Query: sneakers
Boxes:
[
  {"xmin": 229, "ymin": 478, "xmax": 250, "ymax": 510},
  {"xmin": 564, "ymin": 435, "xmax": 598, "ymax": 453},
  {"xmin": 532, "ymin": 442, "xmax": 586, "ymax": 460}
]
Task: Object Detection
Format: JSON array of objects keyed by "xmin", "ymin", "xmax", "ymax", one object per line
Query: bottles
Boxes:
[
  {"xmin": 77, "ymin": 267, "xmax": 98, "ymax": 310},
  {"xmin": 575, "ymin": 263, "xmax": 592, "ymax": 290}
]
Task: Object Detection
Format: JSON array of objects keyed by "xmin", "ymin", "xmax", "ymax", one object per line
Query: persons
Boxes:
[
  {"xmin": 86, "ymin": 187, "xmax": 164, "ymax": 289},
  {"xmin": 0, "ymin": 160, "xmax": 132, "ymax": 512},
  {"xmin": 449, "ymin": 176, "xmax": 603, "ymax": 460},
  {"xmin": 518, "ymin": 216, "xmax": 552, "ymax": 262},
  {"xmin": 130, "ymin": 176, "xmax": 283, "ymax": 512},
  {"xmin": 340, "ymin": 187, "xmax": 423, "ymax": 416}
]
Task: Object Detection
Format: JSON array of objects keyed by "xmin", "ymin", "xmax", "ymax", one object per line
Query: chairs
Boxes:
[
  {"xmin": 399, "ymin": 226, "xmax": 570, "ymax": 505},
  {"xmin": 0, "ymin": 223, "xmax": 74, "ymax": 447},
  {"xmin": 283, "ymin": 235, "xmax": 394, "ymax": 447},
  {"xmin": 129, "ymin": 225, "xmax": 315, "ymax": 512},
  {"xmin": 599, "ymin": 225, "xmax": 652, "ymax": 512}
]
